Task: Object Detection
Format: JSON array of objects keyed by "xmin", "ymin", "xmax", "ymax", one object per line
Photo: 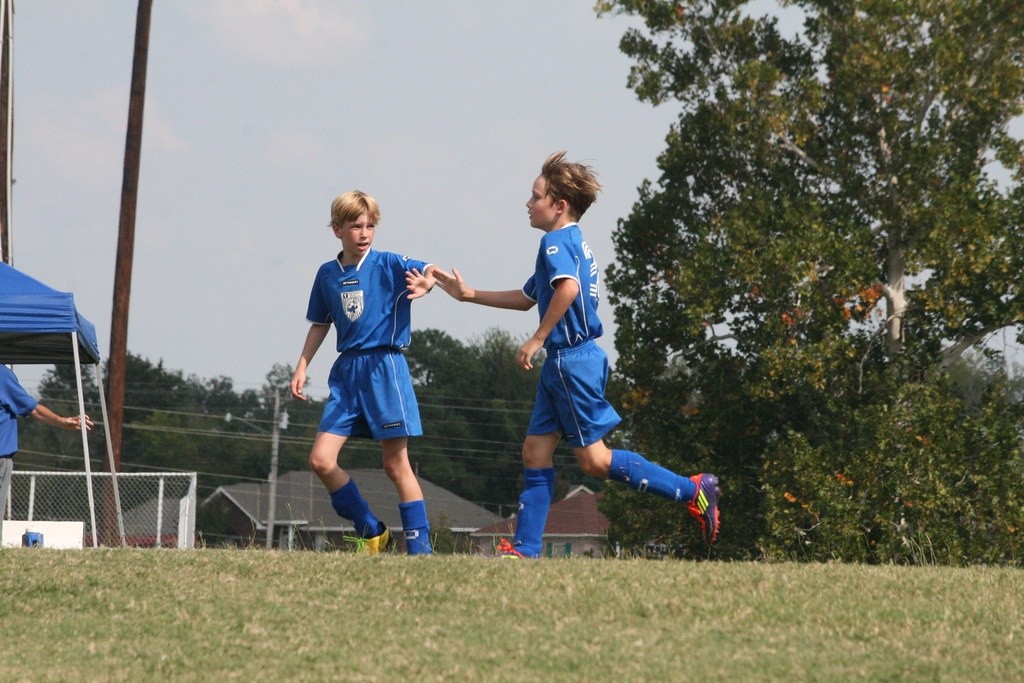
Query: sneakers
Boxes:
[
  {"xmin": 492, "ymin": 552, "xmax": 524, "ymax": 560},
  {"xmin": 683, "ymin": 472, "xmax": 722, "ymax": 547},
  {"xmin": 358, "ymin": 521, "xmax": 395, "ymax": 552}
]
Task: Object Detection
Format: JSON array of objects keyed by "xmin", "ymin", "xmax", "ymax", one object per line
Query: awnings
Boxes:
[{"xmin": 0, "ymin": 261, "xmax": 101, "ymax": 365}]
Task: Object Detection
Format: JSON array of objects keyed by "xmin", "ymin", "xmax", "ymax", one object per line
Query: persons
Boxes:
[
  {"xmin": 288, "ymin": 187, "xmax": 442, "ymax": 562},
  {"xmin": 430, "ymin": 148, "xmax": 722, "ymax": 558},
  {"xmin": 0, "ymin": 362, "xmax": 95, "ymax": 539}
]
